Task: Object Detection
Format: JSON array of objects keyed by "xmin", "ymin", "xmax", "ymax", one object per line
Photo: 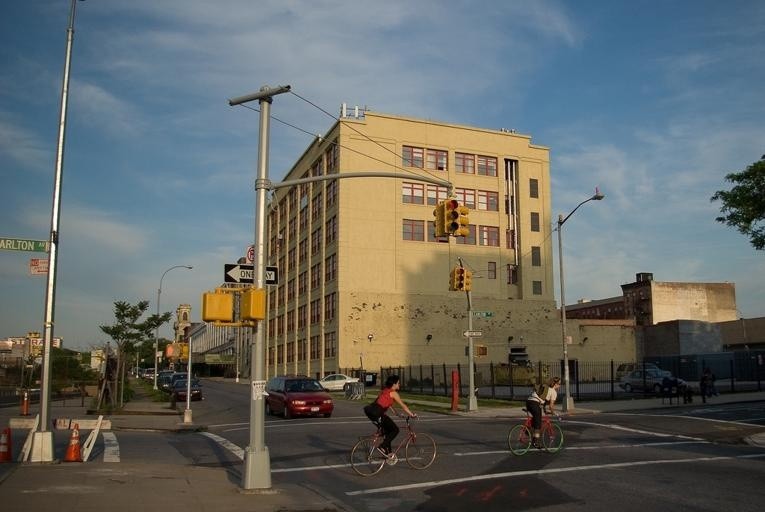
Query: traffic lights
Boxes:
[
  {"xmin": 453, "ymin": 206, "xmax": 470, "ymax": 238},
  {"xmin": 464, "ymin": 268, "xmax": 472, "ymax": 292},
  {"xmin": 477, "ymin": 347, "xmax": 488, "ymax": 355},
  {"xmin": 442, "ymin": 198, "xmax": 462, "ymax": 235},
  {"xmin": 430, "ymin": 203, "xmax": 445, "ymax": 238},
  {"xmin": 166, "ymin": 345, "xmax": 179, "ymax": 357},
  {"xmin": 454, "ymin": 266, "xmax": 465, "ymax": 291},
  {"xmin": 447, "ymin": 268, "xmax": 456, "ymax": 292},
  {"xmin": 183, "ymin": 326, "xmax": 189, "ymax": 341}
]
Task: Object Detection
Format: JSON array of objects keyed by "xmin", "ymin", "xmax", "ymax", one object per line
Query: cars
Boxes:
[
  {"xmin": 617, "ymin": 368, "xmax": 687, "ymax": 396},
  {"xmin": 319, "ymin": 373, "xmax": 360, "ymax": 392},
  {"xmin": 265, "ymin": 373, "xmax": 335, "ymax": 420},
  {"xmin": 130, "ymin": 365, "xmax": 204, "ymax": 402}
]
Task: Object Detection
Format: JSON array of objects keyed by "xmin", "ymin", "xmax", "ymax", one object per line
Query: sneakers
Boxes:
[
  {"xmin": 376, "ymin": 443, "xmax": 390, "ymax": 458},
  {"xmin": 531, "ymin": 436, "xmax": 545, "ymax": 448},
  {"xmin": 525, "ymin": 428, "xmax": 531, "ymax": 436}
]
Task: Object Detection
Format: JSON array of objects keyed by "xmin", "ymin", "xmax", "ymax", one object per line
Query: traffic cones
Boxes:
[
  {"xmin": 60, "ymin": 422, "xmax": 85, "ymax": 463},
  {"xmin": 0, "ymin": 425, "xmax": 14, "ymax": 463}
]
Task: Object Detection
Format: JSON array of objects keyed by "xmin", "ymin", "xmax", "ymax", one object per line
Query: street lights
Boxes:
[
  {"xmin": 557, "ymin": 187, "xmax": 606, "ymax": 411},
  {"xmin": 153, "ymin": 265, "xmax": 193, "ymax": 391}
]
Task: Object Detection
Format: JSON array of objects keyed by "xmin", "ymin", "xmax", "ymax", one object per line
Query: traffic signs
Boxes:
[
  {"xmin": 0, "ymin": 236, "xmax": 49, "ymax": 254},
  {"xmin": 461, "ymin": 330, "xmax": 483, "ymax": 338}
]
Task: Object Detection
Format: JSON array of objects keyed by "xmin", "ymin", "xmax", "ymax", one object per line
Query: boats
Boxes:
[
  {"xmin": 11, "ymin": 385, "xmax": 58, "ymax": 400},
  {"xmin": 57, "ymin": 386, "xmax": 80, "ymax": 397}
]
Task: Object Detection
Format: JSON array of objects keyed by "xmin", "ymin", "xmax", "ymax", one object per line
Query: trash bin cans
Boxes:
[
  {"xmin": 366, "ymin": 372, "xmax": 386, "ymax": 388},
  {"xmin": 20, "ymin": 391, "xmax": 30, "ymax": 415},
  {"xmin": 660, "ymin": 376, "xmax": 679, "ymax": 405}
]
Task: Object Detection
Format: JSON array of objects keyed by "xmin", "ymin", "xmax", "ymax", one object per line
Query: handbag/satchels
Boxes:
[{"xmin": 362, "ymin": 402, "xmax": 386, "ymax": 423}]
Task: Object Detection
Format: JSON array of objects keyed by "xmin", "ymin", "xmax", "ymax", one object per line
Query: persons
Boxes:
[
  {"xmin": 526, "ymin": 377, "xmax": 562, "ymax": 446},
  {"xmin": 661, "ymin": 366, "xmax": 719, "ymax": 405},
  {"xmin": 474, "ymin": 385, "xmax": 478, "ymax": 397},
  {"xmin": 364, "ymin": 375, "xmax": 416, "ymax": 458}
]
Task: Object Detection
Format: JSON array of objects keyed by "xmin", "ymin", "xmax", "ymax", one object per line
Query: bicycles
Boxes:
[
  {"xmin": 506, "ymin": 407, "xmax": 565, "ymax": 457},
  {"xmin": 347, "ymin": 411, "xmax": 438, "ymax": 478}
]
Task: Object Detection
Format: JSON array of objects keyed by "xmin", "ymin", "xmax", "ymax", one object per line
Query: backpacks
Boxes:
[{"xmin": 532, "ymin": 382, "xmax": 550, "ymax": 401}]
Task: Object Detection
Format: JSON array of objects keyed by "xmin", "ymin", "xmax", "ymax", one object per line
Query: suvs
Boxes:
[{"xmin": 613, "ymin": 362, "xmax": 672, "ymax": 383}]
